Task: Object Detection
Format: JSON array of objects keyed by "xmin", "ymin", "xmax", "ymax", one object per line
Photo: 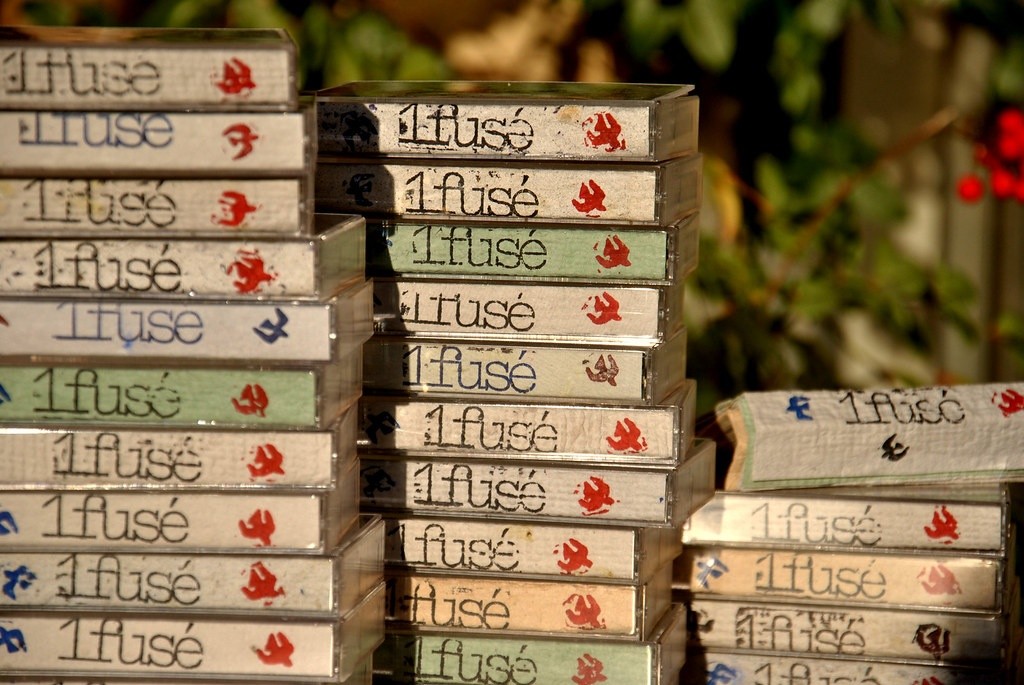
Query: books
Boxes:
[
  {"xmin": 670, "ymin": 480, "xmax": 1016, "ymax": 685},
  {"xmin": 0, "ymin": 25, "xmax": 388, "ymax": 685},
  {"xmin": 313, "ymin": 75, "xmax": 715, "ymax": 685}
]
